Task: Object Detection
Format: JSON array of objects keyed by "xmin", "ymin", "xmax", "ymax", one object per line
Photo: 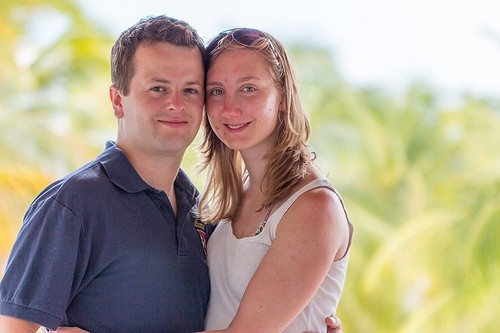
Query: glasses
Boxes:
[{"xmin": 205, "ymin": 28, "xmax": 279, "ymax": 62}]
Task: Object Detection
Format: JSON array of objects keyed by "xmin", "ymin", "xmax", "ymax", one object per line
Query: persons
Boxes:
[
  {"xmin": 1, "ymin": 13, "xmax": 346, "ymax": 333},
  {"xmin": 200, "ymin": 26, "xmax": 351, "ymax": 332}
]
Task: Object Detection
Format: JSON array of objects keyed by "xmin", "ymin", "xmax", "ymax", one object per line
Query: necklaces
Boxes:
[{"xmin": 253, "ymin": 200, "xmax": 277, "ymax": 235}]
